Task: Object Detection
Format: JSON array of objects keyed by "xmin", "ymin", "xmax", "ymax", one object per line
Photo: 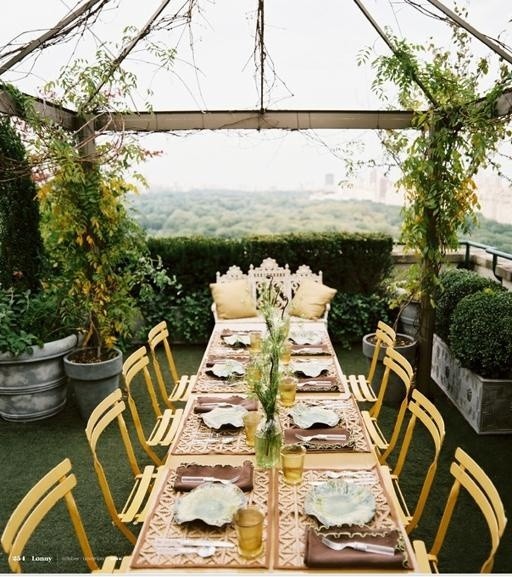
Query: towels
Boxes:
[
  {"xmin": 283, "ymin": 428, "xmax": 354, "ymax": 451},
  {"xmin": 171, "ymin": 460, "xmax": 257, "ymax": 494},
  {"xmin": 302, "ymin": 522, "xmax": 409, "ymax": 570},
  {"xmin": 193, "ymin": 327, "xmax": 340, "ymax": 413}
]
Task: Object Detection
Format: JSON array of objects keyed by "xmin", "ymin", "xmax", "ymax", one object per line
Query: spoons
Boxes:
[{"xmin": 151, "ymin": 547, "xmax": 216, "ymax": 558}]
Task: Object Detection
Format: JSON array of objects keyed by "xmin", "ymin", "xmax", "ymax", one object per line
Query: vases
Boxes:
[{"xmin": 254, "ymin": 426, "xmax": 282, "ymax": 471}]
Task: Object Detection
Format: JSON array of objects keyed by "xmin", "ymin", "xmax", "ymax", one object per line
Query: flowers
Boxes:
[{"xmin": 250, "ymin": 275, "xmax": 289, "ymax": 469}]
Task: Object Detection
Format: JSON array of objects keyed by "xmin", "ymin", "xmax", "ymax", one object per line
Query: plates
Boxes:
[
  {"xmin": 291, "ymin": 362, "xmax": 330, "ymax": 378},
  {"xmin": 205, "ymin": 359, "xmax": 246, "ymax": 378},
  {"xmin": 302, "ymin": 479, "xmax": 378, "ymax": 527},
  {"xmin": 223, "ymin": 335, "xmax": 250, "ymax": 345},
  {"xmin": 195, "ymin": 405, "xmax": 248, "ymax": 430},
  {"xmin": 288, "ymin": 329, "xmax": 324, "ymax": 345},
  {"xmin": 171, "ymin": 481, "xmax": 247, "ymax": 530},
  {"xmin": 290, "ymin": 407, "xmax": 341, "ymax": 428}
]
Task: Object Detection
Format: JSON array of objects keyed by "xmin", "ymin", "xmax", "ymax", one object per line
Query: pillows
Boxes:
[
  {"xmin": 286, "ymin": 281, "xmax": 337, "ymax": 319},
  {"xmin": 209, "ymin": 280, "xmax": 258, "ymax": 319}
]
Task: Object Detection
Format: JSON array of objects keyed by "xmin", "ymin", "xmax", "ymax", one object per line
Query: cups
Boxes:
[
  {"xmin": 230, "ymin": 502, "xmax": 268, "ymax": 560},
  {"xmin": 242, "ymin": 411, "xmax": 265, "ymax": 448},
  {"xmin": 278, "ymin": 375, "xmax": 299, "ymax": 409},
  {"xmin": 281, "ymin": 442, "xmax": 306, "ymax": 486},
  {"xmin": 278, "ymin": 343, "xmax": 294, "ymax": 366},
  {"xmin": 249, "ymin": 332, "xmax": 266, "ymax": 351},
  {"xmin": 246, "ymin": 361, "xmax": 263, "ymax": 391}
]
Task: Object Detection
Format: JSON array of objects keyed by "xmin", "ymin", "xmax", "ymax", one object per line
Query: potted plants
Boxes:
[
  {"xmin": 2, "ymin": 280, "xmax": 86, "ymax": 423},
  {"xmin": 19, "ymin": 112, "xmax": 185, "ymax": 417},
  {"xmin": 429, "ymin": 267, "xmax": 511, "ymax": 435}
]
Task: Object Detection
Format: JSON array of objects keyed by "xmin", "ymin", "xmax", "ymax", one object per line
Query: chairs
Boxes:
[
  {"xmin": 339, "ymin": 320, "xmax": 401, "ymax": 405},
  {"xmin": 395, "ymin": 387, "xmax": 449, "ymax": 534},
  {"xmin": 121, "ymin": 344, "xmax": 191, "ymax": 468},
  {"xmin": 359, "ymin": 346, "xmax": 418, "ymax": 464},
  {"xmin": 85, "ymin": 387, "xmax": 167, "ymax": 545},
  {"xmin": 147, "ymin": 321, "xmax": 200, "ymax": 406},
  {"xmin": 0, "ymin": 457, "xmax": 134, "ymax": 574},
  {"xmin": 414, "ymin": 447, "xmax": 509, "ymax": 572}
]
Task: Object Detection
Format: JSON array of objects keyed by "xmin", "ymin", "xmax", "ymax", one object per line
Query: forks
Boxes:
[
  {"xmin": 294, "ymin": 432, "xmax": 348, "ymax": 444},
  {"xmin": 181, "ymin": 475, "xmax": 240, "ymax": 485},
  {"xmin": 297, "ymin": 381, "xmax": 333, "ymax": 388},
  {"xmin": 321, "ymin": 536, "xmax": 398, "ymax": 558}
]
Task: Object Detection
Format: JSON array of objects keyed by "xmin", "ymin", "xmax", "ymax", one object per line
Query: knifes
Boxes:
[{"xmin": 152, "ymin": 538, "xmax": 235, "ymax": 547}]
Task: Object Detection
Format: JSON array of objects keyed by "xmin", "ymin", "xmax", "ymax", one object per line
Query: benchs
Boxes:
[{"xmin": 210, "ymin": 257, "xmax": 336, "ymax": 324}]
